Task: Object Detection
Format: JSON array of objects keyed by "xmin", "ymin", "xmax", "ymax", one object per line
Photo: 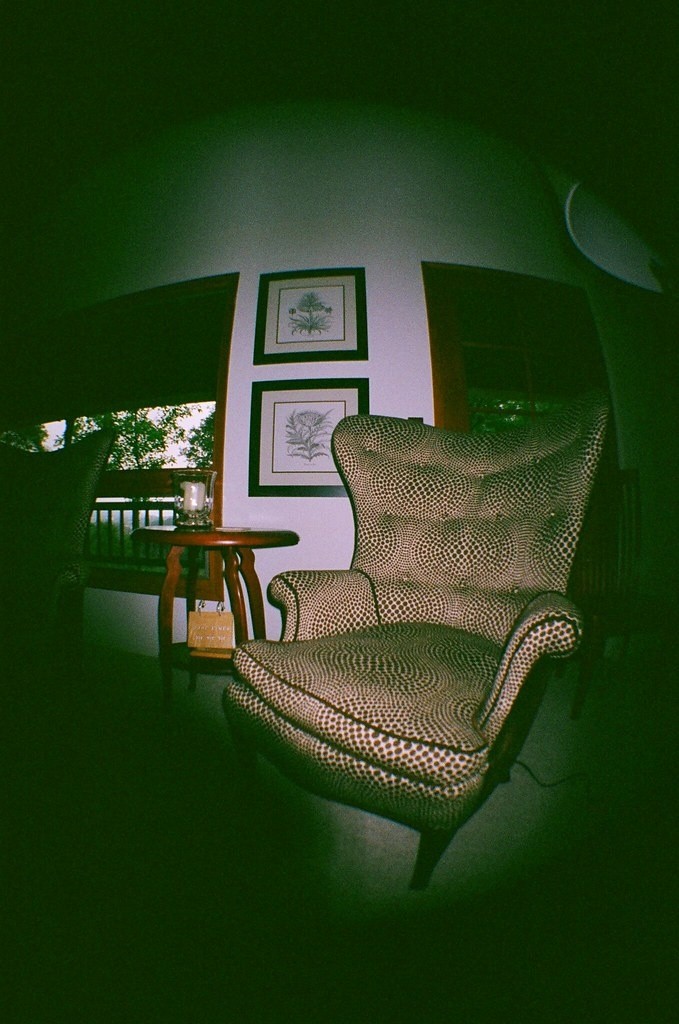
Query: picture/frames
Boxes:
[
  {"xmin": 253, "ymin": 267, "xmax": 369, "ymax": 365},
  {"xmin": 248, "ymin": 378, "xmax": 369, "ymax": 497}
]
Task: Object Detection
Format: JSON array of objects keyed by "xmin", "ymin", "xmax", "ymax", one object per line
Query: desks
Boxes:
[{"xmin": 130, "ymin": 526, "xmax": 299, "ymax": 710}]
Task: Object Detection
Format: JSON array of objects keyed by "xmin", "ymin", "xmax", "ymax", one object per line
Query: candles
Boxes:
[{"xmin": 183, "ymin": 481, "xmax": 205, "ymax": 511}]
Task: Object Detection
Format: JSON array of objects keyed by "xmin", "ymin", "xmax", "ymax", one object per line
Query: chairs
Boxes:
[
  {"xmin": 222, "ymin": 389, "xmax": 610, "ymax": 891},
  {"xmin": 0, "ymin": 428, "xmax": 115, "ymax": 697},
  {"xmin": 567, "ymin": 470, "xmax": 678, "ymax": 715}
]
